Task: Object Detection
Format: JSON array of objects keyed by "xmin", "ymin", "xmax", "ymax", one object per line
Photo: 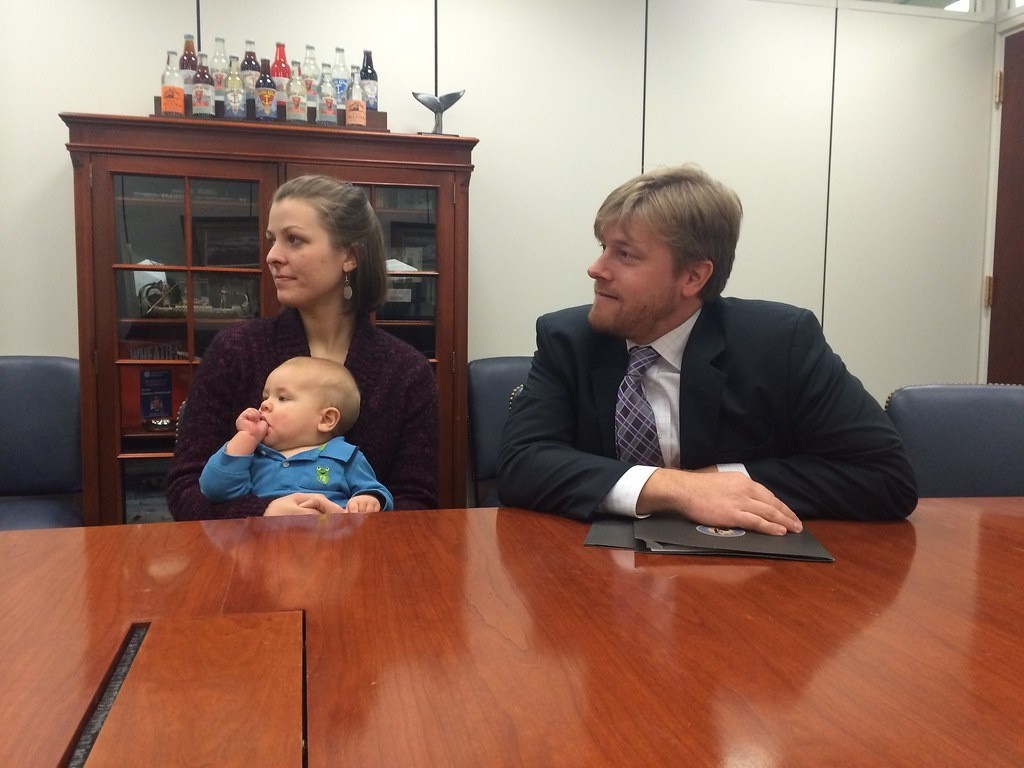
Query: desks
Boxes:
[{"xmin": 0, "ymin": 493, "xmax": 1024, "ymax": 768}]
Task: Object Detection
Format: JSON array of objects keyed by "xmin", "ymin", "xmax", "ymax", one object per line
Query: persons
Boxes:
[
  {"xmin": 199, "ymin": 356, "xmax": 393, "ymax": 514},
  {"xmin": 151, "ymin": 398, "xmax": 163, "ymax": 410},
  {"xmin": 167, "ymin": 175, "xmax": 445, "ymax": 521},
  {"xmin": 498, "ymin": 166, "xmax": 919, "ymax": 536}
]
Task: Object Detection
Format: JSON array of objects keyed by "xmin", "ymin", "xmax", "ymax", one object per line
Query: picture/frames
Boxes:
[
  {"xmin": 177, "ymin": 214, "xmax": 260, "ymax": 268},
  {"xmin": 389, "ymin": 219, "xmax": 437, "ymax": 272}
]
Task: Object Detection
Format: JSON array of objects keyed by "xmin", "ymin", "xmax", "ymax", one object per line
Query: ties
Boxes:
[{"xmin": 614, "ymin": 346, "xmax": 666, "ymax": 468}]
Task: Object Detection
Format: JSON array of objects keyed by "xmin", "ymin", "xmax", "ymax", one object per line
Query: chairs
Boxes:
[
  {"xmin": 884, "ymin": 381, "xmax": 1024, "ymax": 498},
  {"xmin": 0, "ymin": 356, "xmax": 83, "ymax": 533},
  {"xmin": 466, "ymin": 355, "xmax": 532, "ymax": 508}
]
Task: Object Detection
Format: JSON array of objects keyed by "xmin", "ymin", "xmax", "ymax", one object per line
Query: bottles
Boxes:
[
  {"xmin": 301, "ymin": 44, "xmax": 321, "ymax": 107},
  {"xmin": 223, "ymin": 56, "xmax": 247, "ymax": 120},
  {"xmin": 270, "ymin": 42, "xmax": 291, "ymax": 105},
  {"xmin": 286, "ymin": 60, "xmax": 307, "ymax": 123},
  {"xmin": 161, "ymin": 50, "xmax": 185, "ymax": 117},
  {"xmin": 316, "ymin": 63, "xmax": 337, "ymax": 126},
  {"xmin": 210, "ymin": 37, "xmax": 229, "ymax": 101},
  {"xmin": 179, "ymin": 34, "xmax": 198, "ymax": 96},
  {"xmin": 346, "ymin": 65, "xmax": 366, "ymax": 127},
  {"xmin": 255, "ymin": 58, "xmax": 277, "ymax": 121},
  {"xmin": 241, "ymin": 39, "xmax": 260, "ymax": 102},
  {"xmin": 360, "ymin": 50, "xmax": 378, "ymax": 111},
  {"xmin": 332, "ymin": 47, "xmax": 350, "ymax": 110},
  {"xmin": 192, "ymin": 52, "xmax": 215, "ymax": 118}
]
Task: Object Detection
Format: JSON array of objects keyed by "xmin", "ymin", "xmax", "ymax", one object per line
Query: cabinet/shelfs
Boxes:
[{"xmin": 57, "ymin": 111, "xmax": 477, "ymax": 527}]
